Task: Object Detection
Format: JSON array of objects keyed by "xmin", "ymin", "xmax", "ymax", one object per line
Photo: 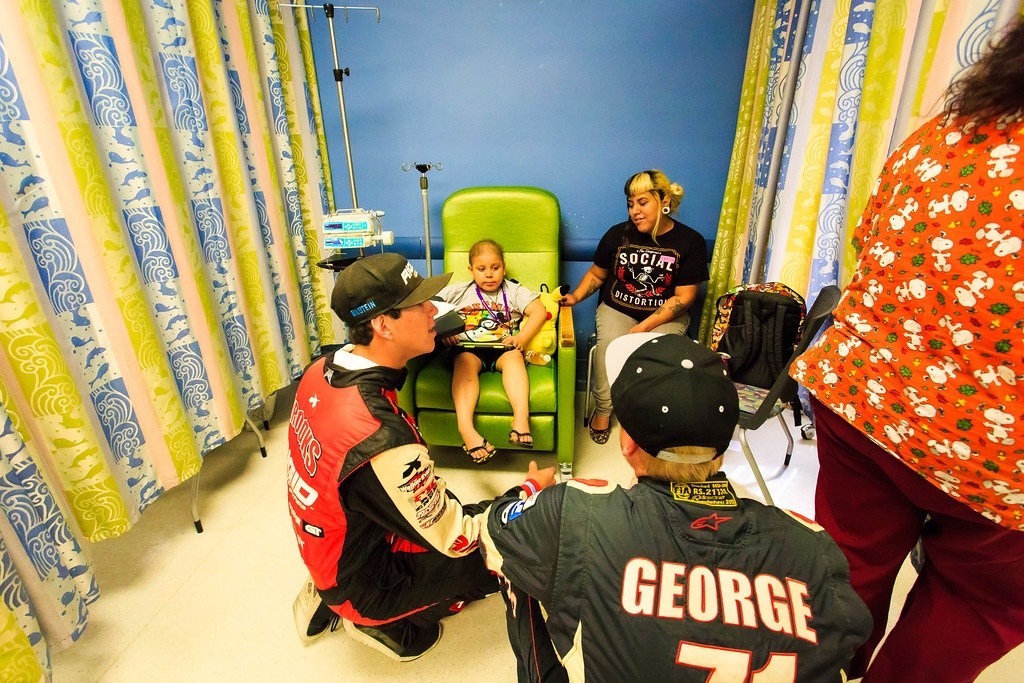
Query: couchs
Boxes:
[{"xmin": 395, "ymin": 186, "xmax": 577, "ymax": 469}]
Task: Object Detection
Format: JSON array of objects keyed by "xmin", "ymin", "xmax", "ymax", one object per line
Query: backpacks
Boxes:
[{"xmin": 710, "ymin": 282, "xmax": 807, "ymax": 427}]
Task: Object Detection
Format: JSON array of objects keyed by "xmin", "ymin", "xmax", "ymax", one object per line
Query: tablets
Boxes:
[{"xmin": 456, "ymin": 342, "xmax": 514, "ymax": 349}]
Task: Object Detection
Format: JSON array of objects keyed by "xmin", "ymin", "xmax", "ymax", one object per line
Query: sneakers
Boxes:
[
  {"xmin": 343, "ymin": 618, "xmax": 442, "ymax": 662},
  {"xmin": 293, "ymin": 573, "xmax": 343, "ymax": 647}
]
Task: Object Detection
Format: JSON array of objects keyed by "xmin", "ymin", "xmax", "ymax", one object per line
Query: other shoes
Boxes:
[{"xmin": 589, "ymin": 410, "xmax": 611, "ymax": 444}]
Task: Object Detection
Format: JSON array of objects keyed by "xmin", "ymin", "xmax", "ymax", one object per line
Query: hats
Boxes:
[
  {"xmin": 331, "ymin": 253, "xmax": 454, "ymax": 327},
  {"xmin": 605, "ymin": 332, "xmax": 740, "ymax": 464}
]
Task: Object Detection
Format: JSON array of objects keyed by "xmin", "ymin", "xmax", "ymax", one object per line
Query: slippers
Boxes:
[
  {"xmin": 462, "ymin": 437, "xmax": 498, "ymax": 464},
  {"xmin": 508, "ymin": 430, "xmax": 534, "ymax": 449}
]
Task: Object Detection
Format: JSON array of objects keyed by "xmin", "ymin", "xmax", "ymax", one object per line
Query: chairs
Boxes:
[{"xmin": 733, "ymin": 284, "xmax": 842, "ymax": 506}]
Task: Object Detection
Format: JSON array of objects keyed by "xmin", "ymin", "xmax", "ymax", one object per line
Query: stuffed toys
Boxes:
[{"xmin": 510, "ymin": 277, "xmax": 570, "ymax": 355}]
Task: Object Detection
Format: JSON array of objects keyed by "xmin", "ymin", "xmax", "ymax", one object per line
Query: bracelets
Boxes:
[{"xmin": 523, "ymin": 481, "xmax": 536, "ymax": 495}]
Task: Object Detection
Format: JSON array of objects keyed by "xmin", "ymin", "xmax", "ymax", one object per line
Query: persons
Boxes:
[
  {"xmin": 287, "ymin": 253, "xmax": 559, "ymax": 662},
  {"xmin": 789, "ymin": 11, "xmax": 1024, "ymax": 683},
  {"xmin": 437, "ymin": 240, "xmax": 547, "ymax": 465},
  {"xmin": 486, "ymin": 332, "xmax": 874, "ymax": 683},
  {"xmin": 559, "ymin": 169, "xmax": 710, "ymax": 445}
]
